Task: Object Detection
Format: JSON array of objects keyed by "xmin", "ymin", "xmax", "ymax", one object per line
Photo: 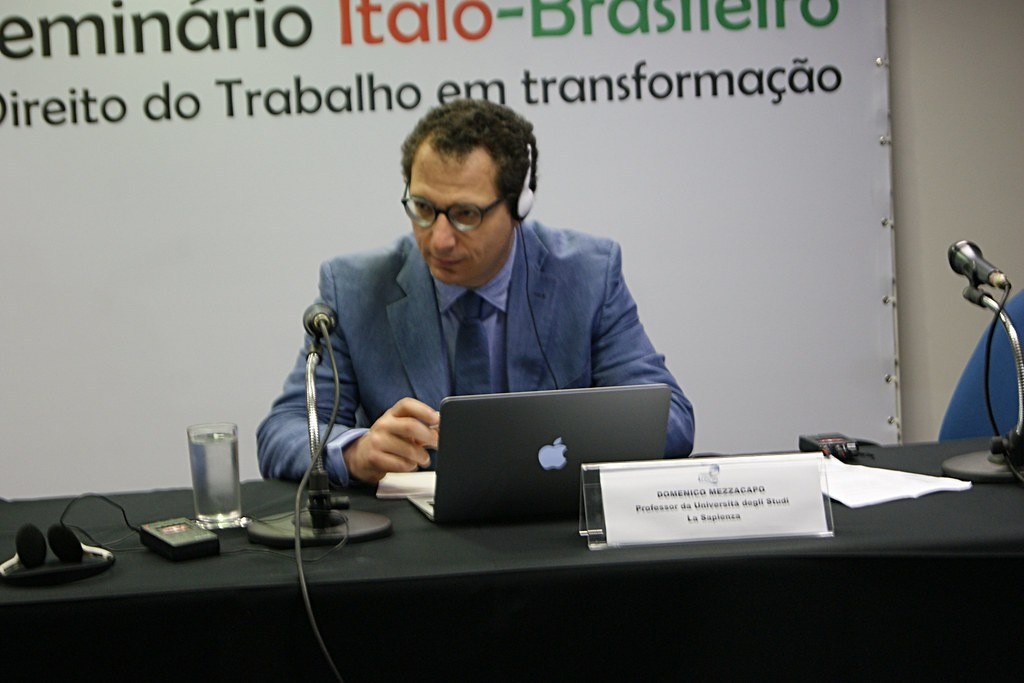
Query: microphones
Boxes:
[
  {"xmin": 302, "ymin": 303, "xmax": 337, "ymax": 337},
  {"xmin": 947, "ymin": 239, "xmax": 1011, "ymax": 290}
]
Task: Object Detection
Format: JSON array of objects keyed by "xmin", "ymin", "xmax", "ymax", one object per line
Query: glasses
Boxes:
[{"xmin": 400, "ymin": 185, "xmax": 505, "ymax": 230}]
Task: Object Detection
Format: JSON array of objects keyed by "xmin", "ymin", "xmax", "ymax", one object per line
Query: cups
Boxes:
[{"xmin": 186, "ymin": 423, "xmax": 242, "ymax": 522}]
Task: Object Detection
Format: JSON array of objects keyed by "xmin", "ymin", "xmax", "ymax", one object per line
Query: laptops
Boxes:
[{"xmin": 407, "ymin": 382, "xmax": 671, "ymax": 524}]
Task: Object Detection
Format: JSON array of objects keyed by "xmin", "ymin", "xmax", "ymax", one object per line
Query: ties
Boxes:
[{"xmin": 451, "ymin": 289, "xmax": 493, "ymax": 396}]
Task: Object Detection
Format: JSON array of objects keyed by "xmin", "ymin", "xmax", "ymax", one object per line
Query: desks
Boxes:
[{"xmin": 0, "ymin": 436, "xmax": 1024, "ymax": 683}]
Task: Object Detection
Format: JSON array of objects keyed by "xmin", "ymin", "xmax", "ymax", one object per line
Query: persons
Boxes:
[{"xmin": 255, "ymin": 99, "xmax": 696, "ymax": 491}]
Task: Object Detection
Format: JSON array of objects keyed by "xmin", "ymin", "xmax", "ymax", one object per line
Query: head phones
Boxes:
[
  {"xmin": 510, "ymin": 142, "xmax": 535, "ymax": 221},
  {"xmin": 0, "ymin": 525, "xmax": 116, "ymax": 587}
]
